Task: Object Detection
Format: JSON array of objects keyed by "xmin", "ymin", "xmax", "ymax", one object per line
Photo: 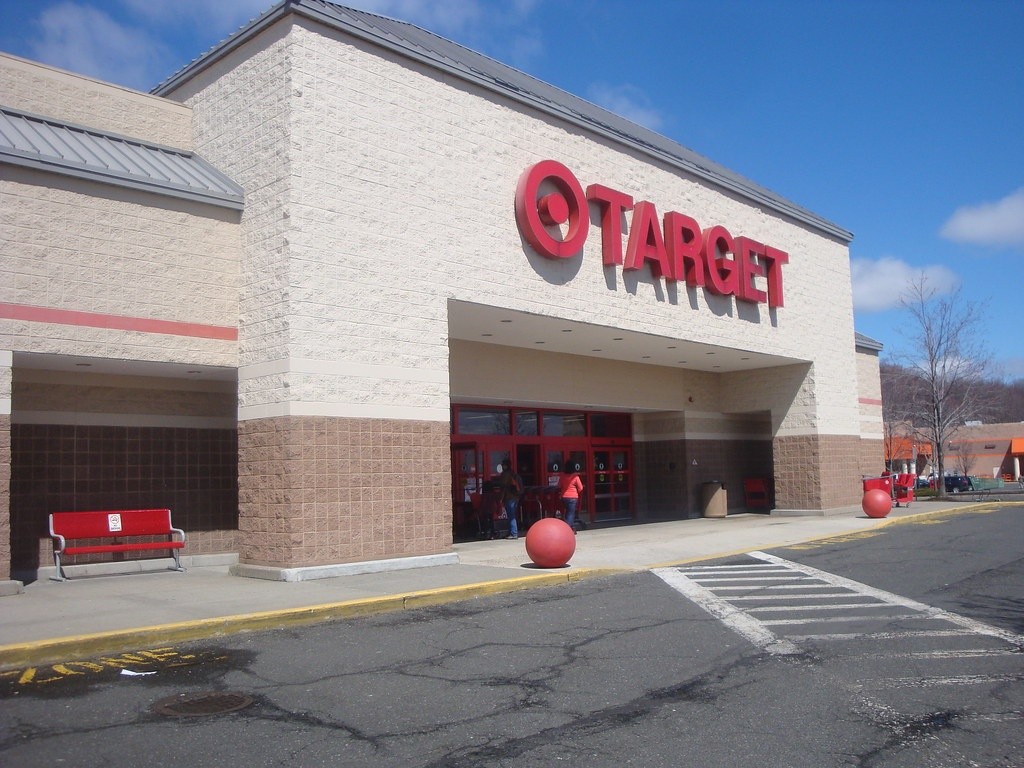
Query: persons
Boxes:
[
  {"xmin": 476, "ymin": 481, "xmax": 502, "ymax": 540},
  {"xmin": 558, "ymin": 461, "xmax": 583, "ymax": 535},
  {"xmin": 497, "ymin": 460, "xmax": 524, "ymax": 539}
]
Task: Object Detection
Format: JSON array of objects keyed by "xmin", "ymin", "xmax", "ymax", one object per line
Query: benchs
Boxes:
[{"xmin": 50, "ymin": 507, "xmax": 187, "ymax": 583}]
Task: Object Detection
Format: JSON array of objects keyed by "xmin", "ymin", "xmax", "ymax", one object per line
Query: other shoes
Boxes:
[{"xmin": 506, "ymin": 533, "xmax": 517, "ymax": 538}]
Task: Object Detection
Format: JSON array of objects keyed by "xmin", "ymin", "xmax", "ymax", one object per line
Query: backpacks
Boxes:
[{"xmin": 504, "ymin": 471, "xmax": 524, "ymax": 495}]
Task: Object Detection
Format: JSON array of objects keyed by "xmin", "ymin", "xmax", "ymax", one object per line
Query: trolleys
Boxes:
[
  {"xmin": 522, "ymin": 486, "xmax": 584, "ymax": 532},
  {"xmin": 465, "ymin": 486, "xmax": 514, "ymax": 539},
  {"xmin": 862, "ymin": 473, "xmax": 915, "ymax": 508},
  {"xmin": 968, "ymin": 476, "xmax": 1005, "ymax": 502}
]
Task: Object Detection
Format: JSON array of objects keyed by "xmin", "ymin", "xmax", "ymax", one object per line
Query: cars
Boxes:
[
  {"xmin": 918, "ymin": 479, "xmax": 930, "ymax": 488},
  {"xmin": 946, "ymin": 475, "xmax": 973, "ymax": 494}
]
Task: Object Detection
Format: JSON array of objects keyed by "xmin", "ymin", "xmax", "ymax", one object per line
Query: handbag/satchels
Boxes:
[{"xmin": 492, "ymin": 496, "xmax": 507, "ymax": 521}]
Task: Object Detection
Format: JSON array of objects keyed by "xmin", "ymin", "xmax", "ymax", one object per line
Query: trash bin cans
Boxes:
[{"xmin": 701, "ymin": 479, "xmax": 728, "ymax": 519}]
